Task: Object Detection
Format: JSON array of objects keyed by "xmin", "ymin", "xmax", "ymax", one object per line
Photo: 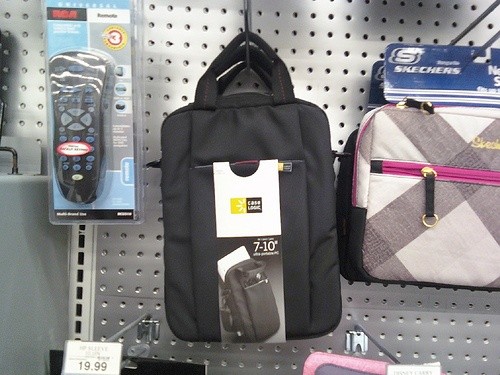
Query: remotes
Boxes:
[{"xmin": 49, "ymin": 54, "xmax": 109, "ymax": 203}]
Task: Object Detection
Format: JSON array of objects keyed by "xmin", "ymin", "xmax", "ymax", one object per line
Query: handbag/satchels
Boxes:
[
  {"xmin": 159, "ymin": 29, "xmax": 342, "ymax": 343},
  {"xmin": 334, "ymin": 96, "xmax": 500, "ymax": 293}
]
